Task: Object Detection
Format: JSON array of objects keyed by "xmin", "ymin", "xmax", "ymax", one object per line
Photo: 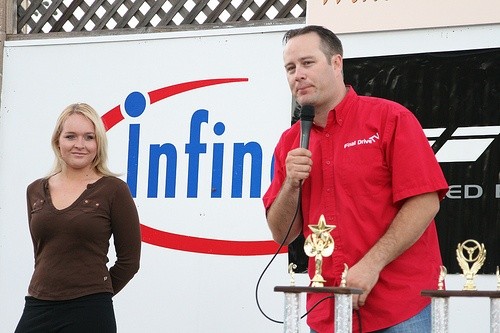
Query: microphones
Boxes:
[{"xmin": 299, "ymin": 105, "xmax": 315, "ymax": 181}]
